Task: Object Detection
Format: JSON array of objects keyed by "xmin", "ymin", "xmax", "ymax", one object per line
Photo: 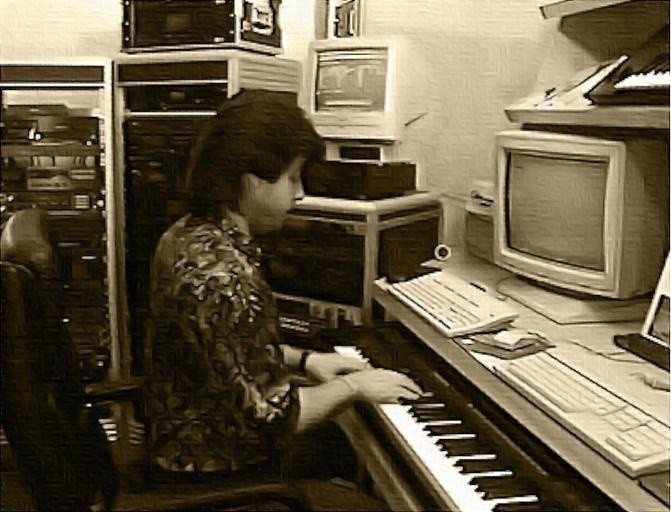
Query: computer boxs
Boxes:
[{"xmin": 300, "ymin": 161, "xmax": 416, "ymax": 200}]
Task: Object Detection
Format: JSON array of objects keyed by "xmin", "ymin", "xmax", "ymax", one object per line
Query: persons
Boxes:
[{"xmin": 140, "ymin": 89, "xmax": 426, "ymax": 507}]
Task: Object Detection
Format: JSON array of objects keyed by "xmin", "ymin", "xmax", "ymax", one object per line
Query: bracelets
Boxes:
[
  {"xmin": 333, "ymin": 375, "xmax": 355, "ymax": 392},
  {"xmin": 299, "ymin": 349, "xmax": 313, "ymax": 373}
]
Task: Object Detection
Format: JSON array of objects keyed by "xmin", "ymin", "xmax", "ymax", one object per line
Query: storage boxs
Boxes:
[{"xmin": 121, "ymin": 0, "xmax": 283, "ymax": 56}]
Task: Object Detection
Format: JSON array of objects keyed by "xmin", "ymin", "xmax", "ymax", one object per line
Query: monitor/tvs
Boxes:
[
  {"xmin": 491, "ymin": 130, "xmax": 670, "ymax": 325},
  {"xmin": 305, "ymin": 37, "xmax": 402, "ymax": 163}
]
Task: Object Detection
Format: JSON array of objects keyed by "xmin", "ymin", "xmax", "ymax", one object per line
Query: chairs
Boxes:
[{"xmin": 0, "ymin": 207, "xmax": 312, "ymax": 512}]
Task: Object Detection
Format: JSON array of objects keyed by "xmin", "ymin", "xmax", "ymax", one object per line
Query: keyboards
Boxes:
[
  {"xmin": 492, "ymin": 349, "xmax": 670, "ymax": 479},
  {"xmin": 387, "ymin": 270, "xmax": 520, "ymax": 339}
]
